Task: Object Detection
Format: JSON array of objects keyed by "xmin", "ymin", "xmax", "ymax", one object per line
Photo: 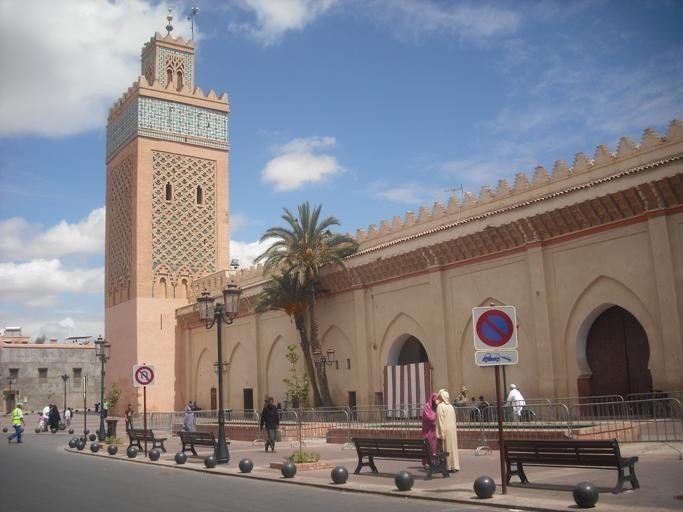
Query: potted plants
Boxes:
[
  {"xmin": 104, "ymin": 379, "xmax": 122, "ymax": 435},
  {"xmin": 282, "ymin": 342, "xmax": 310, "ymax": 409}
]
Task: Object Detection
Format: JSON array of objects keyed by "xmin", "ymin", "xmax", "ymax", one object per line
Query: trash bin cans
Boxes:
[{"xmin": 105, "ymin": 419, "xmax": 119, "ymax": 439}]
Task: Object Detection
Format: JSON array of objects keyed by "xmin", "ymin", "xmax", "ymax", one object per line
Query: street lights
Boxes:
[
  {"xmin": 196, "ymin": 281, "xmax": 242, "ymax": 462},
  {"xmin": 95, "ymin": 338, "xmax": 111, "ymax": 442},
  {"xmin": 61, "ymin": 374, "xmax": 69, "ymax": 418},
  {"xmin": 313, "ymin": 347, "xmax": 334, "ymax": 407}
]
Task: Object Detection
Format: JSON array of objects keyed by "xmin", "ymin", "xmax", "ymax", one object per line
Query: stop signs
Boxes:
[{"xmin": 472, "ymin": 305, "xmax": 518, "ymax": 351}]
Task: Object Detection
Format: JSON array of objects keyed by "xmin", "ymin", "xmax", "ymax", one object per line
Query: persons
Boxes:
[
  {"xmin": 37, "ymin": 404, "xmax": 72, "ymax": 432},
  {"xmin": 96, "ymin": 397, "xmax": 108, "ymax": 419},
  {"xmin": 259, "ymin": 397, "xmax": 282, "ymax": 454},
  {"xmin": 7, "ymin": 402, "xmax": 26, "ymax": 443},
  {"xmin": 435, "ymin": 388, "xmax": 460, "ymax": 473},
  {"xmin": 124, "ymin": 404, "xmax": 133, "ymax": 434},
  {"xmin": 453, "ymin": 383, "xmax": 527, "ymax": 420},
  {"xmin": 422, "ymin": 393, "xmax": 439, "ymax": 470},
  {"xmin": 182, "ymin": 399, "xmax": 198, "ymax": 432}
]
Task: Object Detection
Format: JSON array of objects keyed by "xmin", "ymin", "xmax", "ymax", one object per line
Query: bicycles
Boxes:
[{"xmin": 502, "ymin": 405, "xmax": 535, "ymax": 422}]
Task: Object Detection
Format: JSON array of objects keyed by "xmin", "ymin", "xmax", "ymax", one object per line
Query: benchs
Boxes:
[
  {"xmin": 126, "ymin": 427, "xmax": 230, "ymax": 458},
  {"xmin": 349, "ymin": 437, "xmax": 640, "ymax": 496}
]
